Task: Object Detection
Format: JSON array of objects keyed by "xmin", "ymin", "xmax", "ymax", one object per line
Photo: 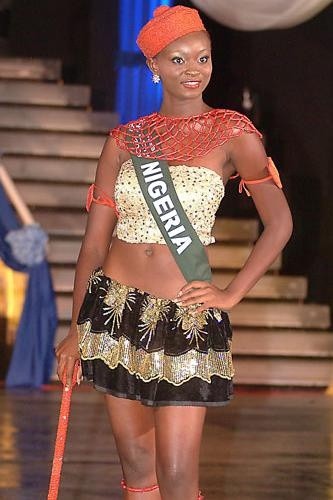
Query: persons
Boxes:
[{"xmin": 56, "ymin": 5, "xmax": 293, "ymax": 500}]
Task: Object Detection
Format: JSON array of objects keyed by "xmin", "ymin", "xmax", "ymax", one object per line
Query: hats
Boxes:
[{"xmin": 136, "ymin": 5, "xmax": 207, "ymax": 60}]
